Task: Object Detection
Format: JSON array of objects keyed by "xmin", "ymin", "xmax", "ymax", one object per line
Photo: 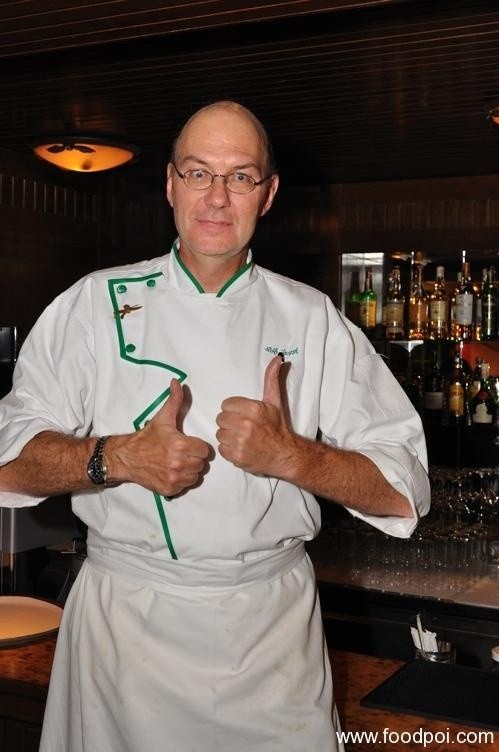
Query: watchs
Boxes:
[{"xmin": 86, "ymin": 432, "xmax": 120, "ymax": 488}]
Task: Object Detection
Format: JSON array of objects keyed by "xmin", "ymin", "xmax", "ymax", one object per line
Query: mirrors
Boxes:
[{"xmin": 339, "ymin": 252, "xmax": 384, "ymax": 324}]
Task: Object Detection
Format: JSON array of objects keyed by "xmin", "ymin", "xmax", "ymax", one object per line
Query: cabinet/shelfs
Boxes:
[{"xmin": 385, "ymin": 251, "xmax": 499, "ymax": 468}]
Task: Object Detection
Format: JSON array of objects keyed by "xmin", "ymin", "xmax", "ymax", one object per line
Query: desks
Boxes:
[{"xmin": 316, "ymin": 562, "xmax": 499, "ymax": 672}]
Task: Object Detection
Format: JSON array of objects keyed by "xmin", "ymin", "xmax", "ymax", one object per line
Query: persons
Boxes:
[{"xmin": 1, "ymin": 100, "xmax": 432, "ymax": 752}]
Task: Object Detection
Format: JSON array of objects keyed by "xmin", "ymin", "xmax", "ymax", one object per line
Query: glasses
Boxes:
[{"xmin": 171, "ymin": 152, "xmax": 274, "ymax": 196}]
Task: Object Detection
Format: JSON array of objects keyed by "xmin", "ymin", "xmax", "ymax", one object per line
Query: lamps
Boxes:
[{"xmin": 28, "ymin": 136, "xmax": 139, "ymax": 171}]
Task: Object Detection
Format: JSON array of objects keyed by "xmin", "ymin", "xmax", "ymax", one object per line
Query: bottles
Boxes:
[
  {"xmin": 427, "ymin": 263, "xmax": 450, "ymax": 341},
  {"xmin": 383, "ymin": 265, "xmax": 405, "ymax": 339},
  {"xmin": 418, "ymin": 342, "xmax": 499, "ymax": 429},
  {"xmin": 407, "ymin": 258, "xmax": 427, "ymax": 340},
  {"xmin": 450, "ymin": 263, "xmax": 475, "ymax": 341},
  {"xmin": 345, "ymin": 271, "xmax": 360, "ymax": 323},
  {"xmin": 475, "ymin": 268, "xmax": 498, "ymax": 342},
  {"xmin": 358, "ymin": 267, "xmax": 379, "ymax": 338}
]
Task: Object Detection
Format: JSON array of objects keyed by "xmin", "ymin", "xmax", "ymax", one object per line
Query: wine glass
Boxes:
[{"xmin": 313, "ymin": 465, "xmax": 498, "ymax": 604}]
